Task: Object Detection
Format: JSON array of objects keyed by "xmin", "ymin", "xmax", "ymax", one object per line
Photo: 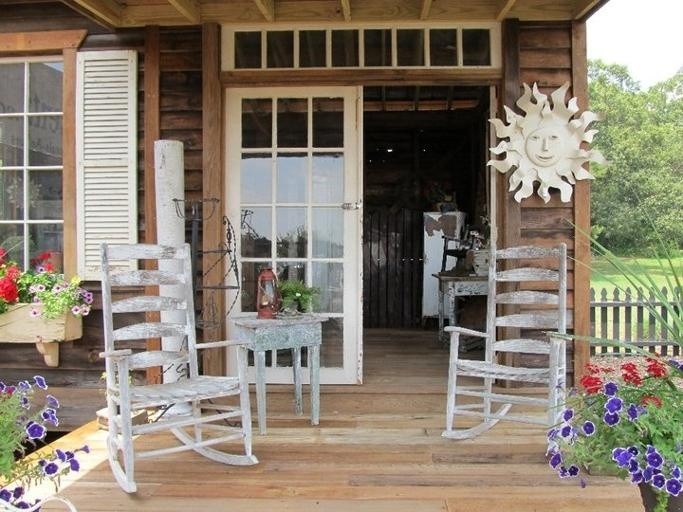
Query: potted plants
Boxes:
[{"xmin": 275, "ymin": 278, "xmax": 320, "ymax": 317}]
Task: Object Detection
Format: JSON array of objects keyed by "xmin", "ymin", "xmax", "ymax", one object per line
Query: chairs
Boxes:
[
  {"xmin": 97, "ymin": 240, "xmax": 259, "ymax": 495},
  {"xmin": 441, "ymin": 240, "xmax": 571, "ymax": 445}
]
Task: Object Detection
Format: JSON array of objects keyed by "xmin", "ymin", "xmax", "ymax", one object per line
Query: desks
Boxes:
[
  {"xmin": 431, "ymin": 272, "xmax": 488, "ymax": 346},
  {"xmin": 227, "ymin": 311, "xmax": 330, "ymax": 436}
]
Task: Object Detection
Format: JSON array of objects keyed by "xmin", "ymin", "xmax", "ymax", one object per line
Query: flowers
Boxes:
[
  {"xmin": 0, "ymin": 243, "xmax": 97, "ymax": 510},
  {"xmin": 546, "ymin": 347, "xmax": 683, "ymax": 499}
]
